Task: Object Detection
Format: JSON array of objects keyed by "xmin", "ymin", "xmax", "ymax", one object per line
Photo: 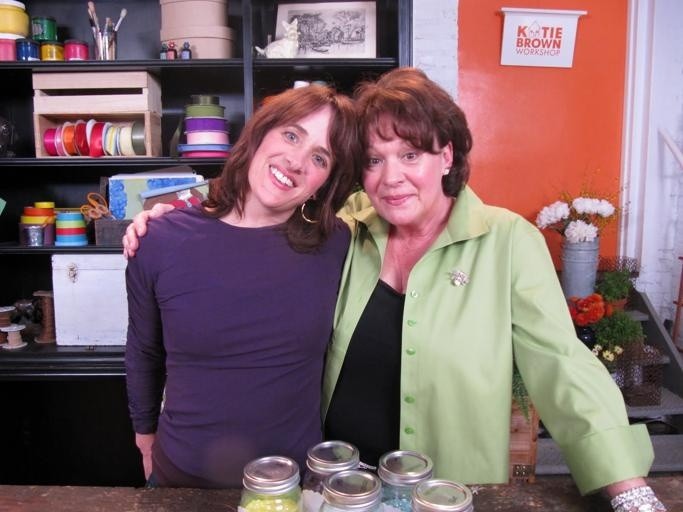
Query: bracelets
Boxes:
[{"xmin": 610, "ymin": 486, "xmax": 667, "ymax": 512}]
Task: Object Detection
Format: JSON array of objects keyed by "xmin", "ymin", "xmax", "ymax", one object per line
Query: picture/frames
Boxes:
[{"xmin": 275, "ymin": 2, "xmax": 377, "ymax": 59}]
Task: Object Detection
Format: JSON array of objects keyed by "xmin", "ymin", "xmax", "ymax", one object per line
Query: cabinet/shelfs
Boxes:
[{"xmin": 0, "ymin": 0, "xmax": 413, "ymax": 489}]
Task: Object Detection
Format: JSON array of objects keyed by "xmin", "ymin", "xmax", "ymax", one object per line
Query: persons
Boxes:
[
  {"xmin": 124, "ymin": 83, "xmax": 353, "ymax": 487},
  {"xmin": 119, "ymin": 66, "xmax": 671, "ymax": 512}
]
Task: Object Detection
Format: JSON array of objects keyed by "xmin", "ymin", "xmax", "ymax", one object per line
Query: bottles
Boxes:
[{"xmin": 233, "ymin": 434, "xmax": 478, "ymax": 512}]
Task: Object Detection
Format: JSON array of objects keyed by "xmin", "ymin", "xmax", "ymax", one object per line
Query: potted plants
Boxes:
[
  {"xmin": 510, "ymin": 363, "xmax": 539, "ymax": 486},
  {"xmin": 591, "ymin": 310, "xmax": 646, "ymax": 390},
  {"xmin": 596, "ymin": 267, "xmax": 639, "ymax": 317}
]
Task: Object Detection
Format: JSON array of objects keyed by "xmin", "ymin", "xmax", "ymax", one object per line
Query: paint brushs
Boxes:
[{"xmin": 87, "ymin": 1, "xmax": 128, "ymax": 61}]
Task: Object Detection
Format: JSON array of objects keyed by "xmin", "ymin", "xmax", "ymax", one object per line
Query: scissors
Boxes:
[{"xmin": 80, "ymin": 192, "xmax": 117, "ymax": 221}]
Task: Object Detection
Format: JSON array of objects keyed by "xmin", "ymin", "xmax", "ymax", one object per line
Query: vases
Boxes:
[
  {"xmin": 576, "ymin": 326, "xmax": 596, "ymax": 351},
  {"xmin": 560, "ymin": 237, "xmax": 600, "ymax": 304}
]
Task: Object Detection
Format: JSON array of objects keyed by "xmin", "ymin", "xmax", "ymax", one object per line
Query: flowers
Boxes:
[
  {"xmin": 566, "ymin": 294, "xmax": 614, "ymax": 328},
  {"xmin": 532, "ymin": 160, "xmax": 637, "ymax": 245}
]
